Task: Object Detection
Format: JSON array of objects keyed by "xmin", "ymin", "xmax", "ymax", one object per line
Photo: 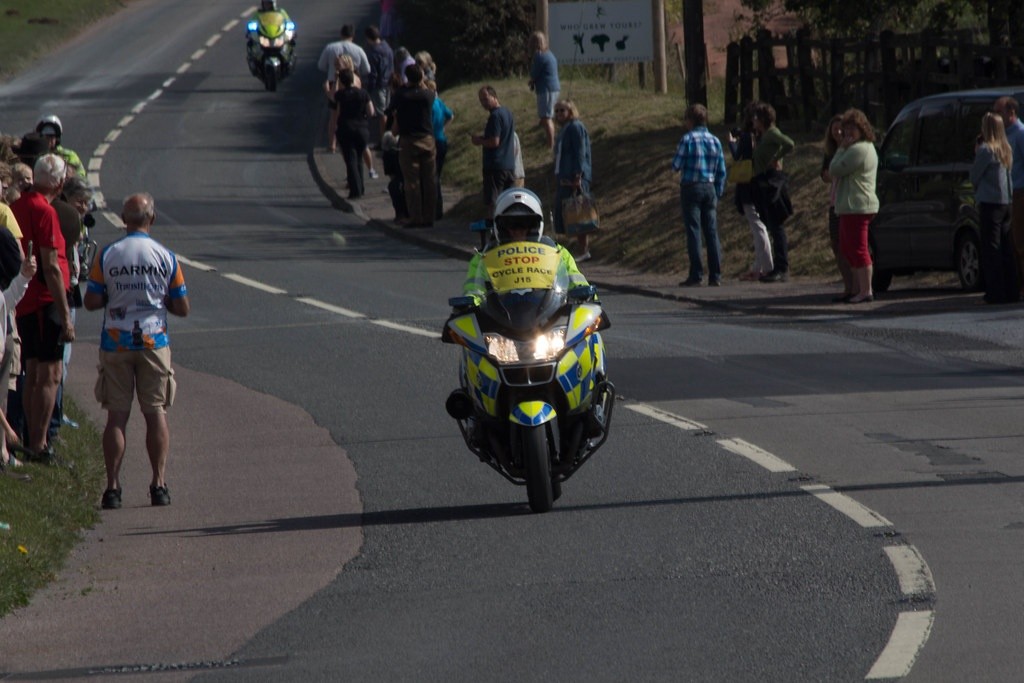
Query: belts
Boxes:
[{"xmin": 681, "ymin": 181, "xmax": 715, "ymax": 188}]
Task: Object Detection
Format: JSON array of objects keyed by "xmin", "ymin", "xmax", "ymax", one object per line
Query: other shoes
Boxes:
[
  {"xmin": 841, "ymin": 293, "xmax": 860, "ymax": 301},
  {"xmin": 707, "ymin": 277, "xmax": 719, "ymax": 286},
  {"xmin": 0, "ymin": 408, "xmax": 81, "ymax": 480},
  {"xmin": 593, "ymin": 371, "xmax": 609, "ymax": 408},
  {"xmin": 150, "ymin": 483, "xmax": 171, "ymax": 506},
  {"xmin": 680, "ymin": 278, "xmax": 702, "ymax": 286},
  {"xmin": 340, "ymin": 165, "xmax": 450, "ymax": 231},
  {"xmin": 447, "ymin": 389, "xmax": 477, "ymax": 420},
  {"xmin": 1007, "ymin": 291, "xmax": 1020, "ymax": 303},
  {"xmin": 848, "ymin": 293, "xmax": 874, "ymax": 302},
  {"xmin": 571, "ymin": 250, "xmax": 591, "ymax": 263},
  {"xmin": 100, "ymin": 487, "xmax": 122, "ymax": 509},
  {"xmin": 990, "ymin": 292, "xmax": 1006, "ymax": 304},
  {"xmin": 759, "ymin": 270, "xmax": 783, "ymax": 283}
]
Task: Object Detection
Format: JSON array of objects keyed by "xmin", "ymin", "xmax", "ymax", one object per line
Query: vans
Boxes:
[{"xmin": 868, "ymin": 86, "xmax": 1024, "ymax": 297}]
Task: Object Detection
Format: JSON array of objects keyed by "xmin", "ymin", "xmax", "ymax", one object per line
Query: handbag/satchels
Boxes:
[{"xmin": 562, "ymin": 182, "xmax": 600, "ymax": 236}]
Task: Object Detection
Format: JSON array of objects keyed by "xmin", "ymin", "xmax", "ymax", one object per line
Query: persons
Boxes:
[
  {"xmin": 552, "ymin": 100, "xmax": 592, "ymax": 261},
  {"xmin": 727, "ymin": 99, "xmax": 795, "ymax": 281},
  {"xmin": 0, "ymin": 114, "xmax": 106, "ymax": 483},
  {"xmin": 471, "ymin": 87, "xmax": 525, "ymax": 216},
  {"xmin": 83, "ymin": 193, "xmax": 190, "ymax": 509},
  {"xmin": 820, "ymin": 107, "xmax": 879, "ymax": 303},
  {"xmin": 318, "ymin": 0, "xmax": 454, "ymax": 228},
  {"xmin": 671, "ymin": 104, "xmax": 726, "ymax": 286},
  {"xmin": 245, "ymin": 0, "xmax": 296, "ymax": 66},
  {"xmin": 969, "ymin": 97, "xmax": 1024, "ymax": 307},
  {"xmin": 527, "ymin": 32, "xmax": 560, "ymax": 153},
  {"xmin": 462, "ymin": 188, "xmax": 597, "ymax": 303}
]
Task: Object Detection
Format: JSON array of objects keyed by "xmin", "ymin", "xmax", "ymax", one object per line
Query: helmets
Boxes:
[
  {"xmin": 492, "ymin": 189, "xmax": 545, "ymax": 245},
  {"xmin": 261, "ymin": 0, "xmax": 277, "ymax": 10},
  {"xmin": 36, "ymin": 115, "xmax": 64, "ymax": 146}
]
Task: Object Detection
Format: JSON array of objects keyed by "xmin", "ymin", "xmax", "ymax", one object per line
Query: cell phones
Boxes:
[{"xmin": 28, "ymin": 241, "xmax": 33, "ymax": 261}]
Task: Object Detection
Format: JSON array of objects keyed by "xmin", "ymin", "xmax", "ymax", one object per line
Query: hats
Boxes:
[{"xmin": 10, "ymin": 134, "xmax": 48, "ymax": 159}]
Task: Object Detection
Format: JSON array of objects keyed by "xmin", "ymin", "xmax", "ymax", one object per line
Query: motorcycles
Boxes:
[
  {"xmin": 441, "ymin": 281, "xmax": 614, "ymax": 511},
  {"xmin": 245, "ymin": 12, "xmax": 298, "ymax": 92}
]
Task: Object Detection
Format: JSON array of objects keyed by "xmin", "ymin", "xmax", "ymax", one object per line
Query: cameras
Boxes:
[{"xmin": 732, "ymin": 127, "xmax": 744, "ymax": 138}]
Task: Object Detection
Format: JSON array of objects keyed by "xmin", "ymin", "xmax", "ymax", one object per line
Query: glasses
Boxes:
[{"xmin": 553, "ymin": 107, "xmax": 567, "ymax": 114}]
